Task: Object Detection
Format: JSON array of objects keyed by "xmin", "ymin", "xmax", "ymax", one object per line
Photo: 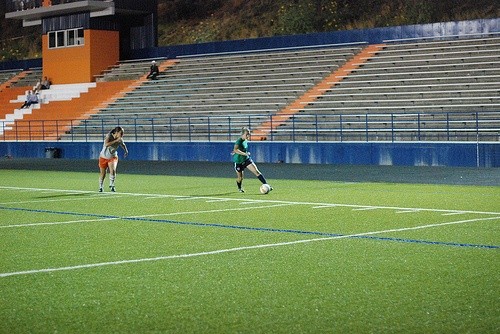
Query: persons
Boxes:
[
  {"xmin": 98, "ymin": 126, "xmax": 129, "ymax": 192},
  {"xmin": 146, "ymin": 61, "xmax": 159, "ymax": 80},
  {"xmin": 232, "ymin": 126, "xmax": 273, "ymax": 193},
  {"xmin": 21, "ymin": 77, "xmax": 50, "ymax": 109}
]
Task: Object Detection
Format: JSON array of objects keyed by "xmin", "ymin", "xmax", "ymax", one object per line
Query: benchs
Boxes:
[{"xmin": 0, "ymin": 32, "xmax": 500, "ymax": 141}]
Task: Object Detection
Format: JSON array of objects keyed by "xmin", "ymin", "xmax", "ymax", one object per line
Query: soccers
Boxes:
[{"xmin": 260, "ymin": 184, "xmax": 270, "ymax": 194}]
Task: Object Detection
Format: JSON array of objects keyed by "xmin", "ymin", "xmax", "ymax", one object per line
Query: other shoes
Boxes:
[
  {"xmin": 99, "ymin": 188, "xmax": 103, "ymax": 193},
  {"xmin": 238, "ymin": 189, "xmax": 244, "ymax": 193},
  {"xmin": 109, "ymin": 185, "xmax": 116, "ymax": 193}
]
